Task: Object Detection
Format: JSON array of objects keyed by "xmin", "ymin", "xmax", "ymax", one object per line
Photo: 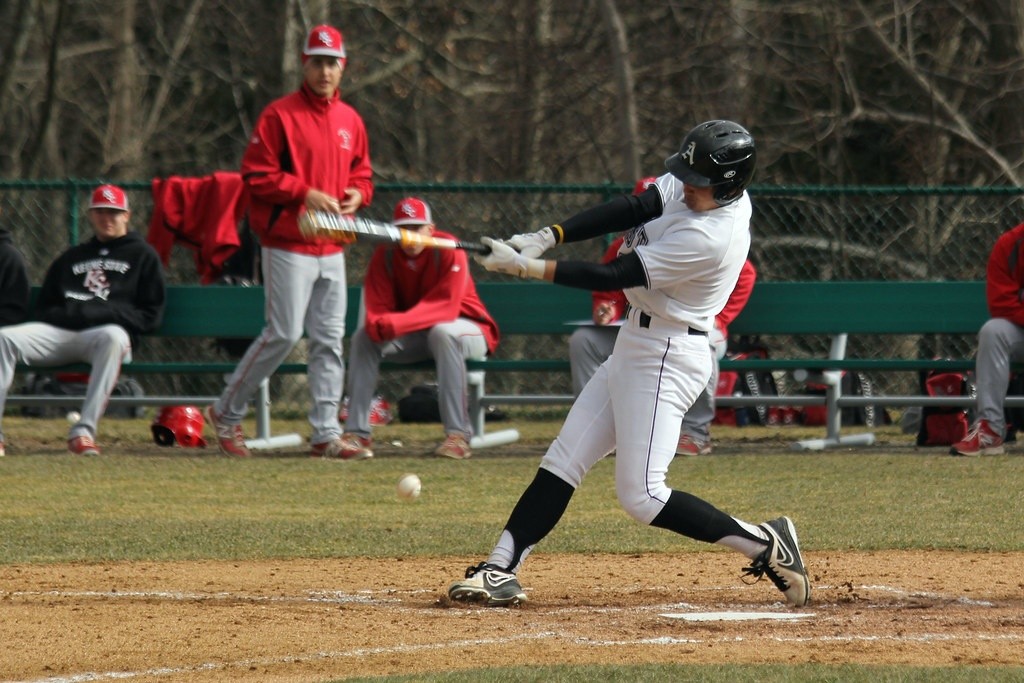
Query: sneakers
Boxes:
[
  {"xmin": 435, "ymin": 431, "xmax": 473, "ymax": 460},
  {"xmin": 205, "ymin": 405, "xmax": 252, "ymax": 458},
  {"xmin": 950, "ymin": 421, "xmax": 1004, "ymax": 457},
  {"xmin": 341, "ymin": 429, "xmax": 372, "ymax": 446},
  {"xmin": 311, "ymin": 435, "xmax": 374, "ymax": 460},
  {"xmin": 367, "ymin": 395, "xmax": 390, "ymax": 426},
  {"xmin": 448, "ymin": 561, "xmax": 528, "ymax": 606},
  {"xmin": 677, "ymin": 433, "xmax": 712, "ymax": 457},
  {"xmin": 739, "ymin": 515, "xmax": 809, "ymax": 608},
  {"xmin": 68, "ymin": 436, "xmax": 98, "ymax": 456}
]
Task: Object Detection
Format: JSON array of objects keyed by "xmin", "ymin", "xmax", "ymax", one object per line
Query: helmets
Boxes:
[
  {"xmin": 663, "ymin": 120, "xmax": 756, "ymax": 206},
  {"xmin": 150, "ymin": 404, "xmax": 206, "ymax": 449}
]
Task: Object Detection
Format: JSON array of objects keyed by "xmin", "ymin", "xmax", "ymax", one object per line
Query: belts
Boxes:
[{"xmin": 626, "ymin": 306, "xmax": 707, "ymax": 336}]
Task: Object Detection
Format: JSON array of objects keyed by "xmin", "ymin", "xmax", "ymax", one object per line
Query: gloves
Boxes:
[
  {"xmin": 505, "ymin": 227, "xmax": 558, "ymax": 260},
  {"xmin": 472, "ymin": 234, "xmax": 547, "ymax": 280}
]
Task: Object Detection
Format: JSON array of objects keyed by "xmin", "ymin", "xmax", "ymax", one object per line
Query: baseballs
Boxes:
[{"xmin": 396, "ymin": 472, "xmax": 422, "ymax": 500}]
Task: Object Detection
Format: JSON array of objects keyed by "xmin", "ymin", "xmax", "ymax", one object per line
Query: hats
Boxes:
[
  {"xmin": 392, "ymin": 197, "xmax": 432, "ymax": 226},
  {"xmin": 88, "ymin": 184, "xmax": 128, "ymax": 210},
  {"xmin": 301, "ymin": 24, "xmax": 348, "ymax": 73}
]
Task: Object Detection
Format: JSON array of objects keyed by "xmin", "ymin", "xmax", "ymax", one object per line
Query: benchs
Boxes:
[{"xmin": 8, "ymin": 283, "xmax": 1024, "ymax": 453}]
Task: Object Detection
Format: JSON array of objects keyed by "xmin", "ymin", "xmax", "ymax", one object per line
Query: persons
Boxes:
[
  {"xmin": 568, "ymin": 176, "xmax": 756, "ymax": 456},
  {"xmin": 949, "ymin": 220, "xmax": 1024, "ymax": 456},
  {"xmin": 449, "ymin": 118, "xmax": 810, "ymax": 607},
  {"xmin": 0, "ymin": 226, "xmax": 29, "ymax": 327},
  {"xmin": 0, "ymin": 183, "xmax": 171, "ymax": 457},
  {"xmin": 204, "ymin": 27, "xmax": 373, "ymax": 461},
  {"xmin": 343, "ymin": 194, "xmax": 499, "ymax": 459}
]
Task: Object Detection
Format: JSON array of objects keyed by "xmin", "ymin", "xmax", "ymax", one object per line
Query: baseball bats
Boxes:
[{"xmin": 298, "ymin": 210, "xmax": 520, "ymax": 258}]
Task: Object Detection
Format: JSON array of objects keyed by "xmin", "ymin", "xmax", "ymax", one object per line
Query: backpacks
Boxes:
[{"xmin": 916, "ymin": 357, "xmax": 978, "ymax": 446}]
